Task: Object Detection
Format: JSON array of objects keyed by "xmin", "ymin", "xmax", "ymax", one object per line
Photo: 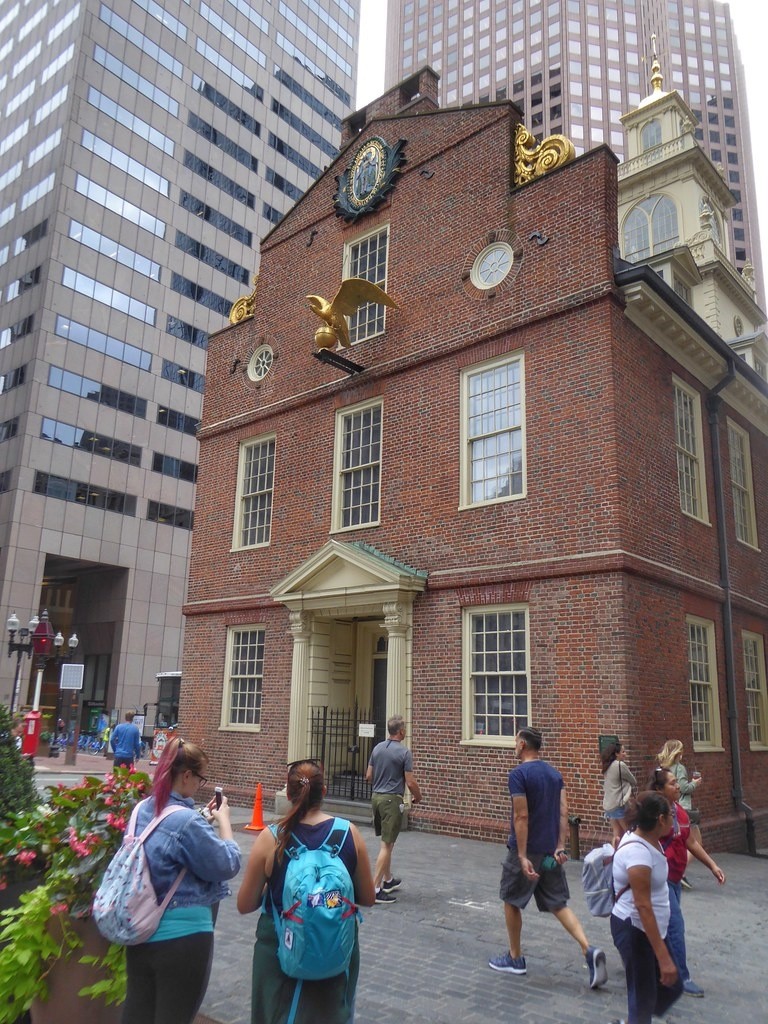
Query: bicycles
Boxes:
[{"xmin": 49, "ymin": 729, "xmax": 107, "ymax": 756}]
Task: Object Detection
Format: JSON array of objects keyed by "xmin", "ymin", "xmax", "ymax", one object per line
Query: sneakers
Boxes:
[
  {"xmin": 681, "ymin": 877, "xmax": 692, "ymax": 889},
  {"xmin": 383, "ymin": 877, "xmax": 402, "ymax": 892},
  {"xmin": 683, "ymin": 979, "xmax": 704, "ymax": 997},
  {"xmin": 585, "ymin": 945, "xmax": 608, "ymax": 988},
  {"xmin": 375, "ymin": 888, "xmax": 397, "ymax": 904},
  {"xmin": 488, "ymin": 949, "xmax": 526, "ymax": 975}
]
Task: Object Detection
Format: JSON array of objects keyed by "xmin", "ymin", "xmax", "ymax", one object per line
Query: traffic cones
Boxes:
[{"xmin": 245, "ymin": 783, "xmax": 266, "ymax": 830}]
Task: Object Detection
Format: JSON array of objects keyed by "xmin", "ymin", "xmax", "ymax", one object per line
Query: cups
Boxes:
[{"xmin": 692, "ymin": 772, "xmax": 701, "ymax": 780}]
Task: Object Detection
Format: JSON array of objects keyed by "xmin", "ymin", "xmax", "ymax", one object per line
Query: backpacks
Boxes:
[
  {"xmin": 261, "ymin": 817, "xmax": 362, "ymax": 981},
  {"xmin": 93, "ymin": 799, "xmax": 189, "ymax": 946},
  {"xmin": 581, "ymin": 836, "xmax": 652, "ymax": 917}
]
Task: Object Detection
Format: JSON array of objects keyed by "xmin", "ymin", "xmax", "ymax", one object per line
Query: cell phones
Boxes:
[{"xmin": 215, "ymin": 787, "xmax": 224, "ymax": 810}]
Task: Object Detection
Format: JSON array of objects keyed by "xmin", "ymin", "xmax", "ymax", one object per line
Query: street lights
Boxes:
[
  {"xmin": 28, "ymin": 609, "xmax": 56, "ymax": 710},
  {"xmin": 6, "ymin": 609, "xmax": 40, "ymax": 721},
  {"xmin": 49, "ymin": 631, "xmax": 86, "ymax": 758}
]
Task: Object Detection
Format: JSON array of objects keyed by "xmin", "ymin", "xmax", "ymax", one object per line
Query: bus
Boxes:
[{"xmin": 148, "ymin": 668, "xmax": 182, "ymax": 765}]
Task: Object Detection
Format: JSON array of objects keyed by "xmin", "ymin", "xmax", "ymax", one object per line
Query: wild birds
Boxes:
[{"xmin": 305, "ymin": 277, "xmax": 399, "ymax": 349}]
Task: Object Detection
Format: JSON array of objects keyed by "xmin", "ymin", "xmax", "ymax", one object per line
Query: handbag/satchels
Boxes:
[{"xmin": 685, "ymin": 809, "xmax": 700, "ymax": 825}]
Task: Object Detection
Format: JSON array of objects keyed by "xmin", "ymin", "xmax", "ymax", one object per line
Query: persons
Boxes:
[
  {"xmin": 601, "ymin": 742, "xmax": 639, "ymax": 847},
  {"xmin": 647, "ymin": 766, "xmax": 726, "ymax": 997},
  {"xmin": 237, "ymin": 758, "xmax": 376, "ymax": 1024},
  {"xmin": 488, "ymin": 726, "xmax": 610, "ymax": 989},
  {"xmin": 659, "ymin": 737, "xmax": 703, "ymax": 889},
  {"xmin": 610, "ymin": 789, "xmax": 685, "ymax": 1024},
  {"xmin": 120, "ymin": 737, "xmax": 243, "ymax": 1024},
  {"xmin": 110, "ymin": 711, "xmax": 141, "ymax": 774},
  {"xmin": 12, "ymin": 718, "xmax": 32, "ymax": 759},
  {"xmin": 367, "ymin": 715, "xmax": 423, "ymax": 905}
]
